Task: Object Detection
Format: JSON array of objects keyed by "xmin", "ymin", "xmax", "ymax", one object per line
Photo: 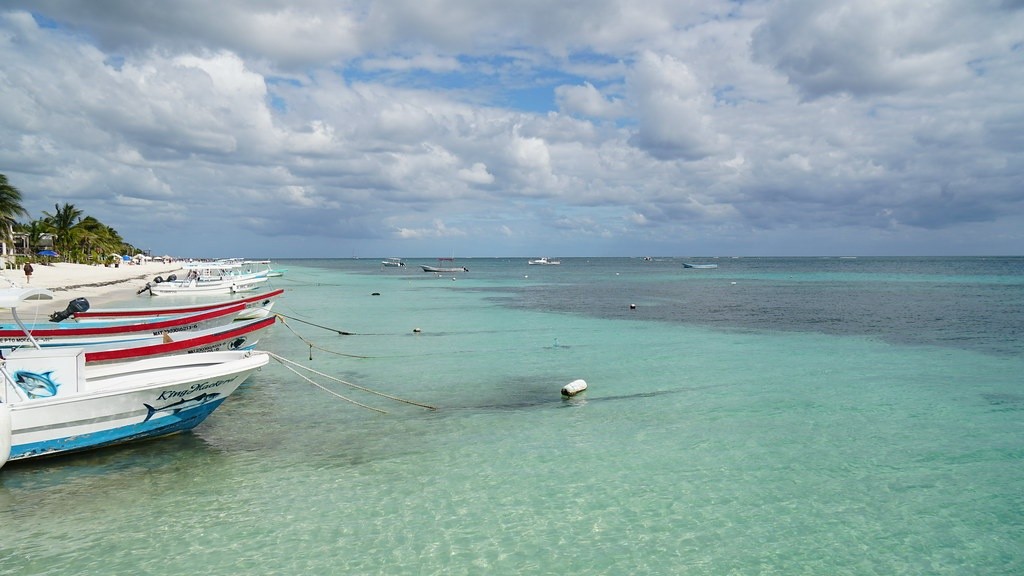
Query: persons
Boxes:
[{"xmin": 24, "ymin": 262, "xmax": 33, "ymax": 283}]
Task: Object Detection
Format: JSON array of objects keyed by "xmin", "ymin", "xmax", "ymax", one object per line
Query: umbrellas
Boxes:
[
  {"xmin": 123, "ymin": 254, "xmax": 162, "ymax": 263},
  {"xmin": 37, "ymin": 250, "xmax": 60, "ymax": 266}
]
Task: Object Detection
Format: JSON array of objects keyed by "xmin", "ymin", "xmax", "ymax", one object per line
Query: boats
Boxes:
[
  {"xmin": 681, "ymin": 261, "xmax": 718, "ymax": 269},
  {"xmin": 0, "ymin": 302, "xmax": 247, "ymax": 342},
  {"xmin": 48, "ymin": 286, "xmax": 285, "ymax": 321},
  {"xmin": 0, "ymin": 288, "xmax": 269, "ymax": 469},
  {"xmin": 644, "ymin": 256, "xmax": 652, "ymax": 261},
  {"xmin": 382, "ymin": 257, "xmax": 405, "ymax": 267},
  {"xmin": 420, "ymin": 257, "xmax": 469, "ymax": 272},
  {"xmin": 84, "ymin": 315, "xmax": 277, "ymax": 366},
  {"xmin": 528, "ymin": 257, "xmax": 561, "ymax": 265},
  {"xmin": 438, "ymin": 256, "xmax": 456, "ymax": 262},
  {"xmin": 137, "ymin": 258, "xmax": 289, "ymax": 296}
]
